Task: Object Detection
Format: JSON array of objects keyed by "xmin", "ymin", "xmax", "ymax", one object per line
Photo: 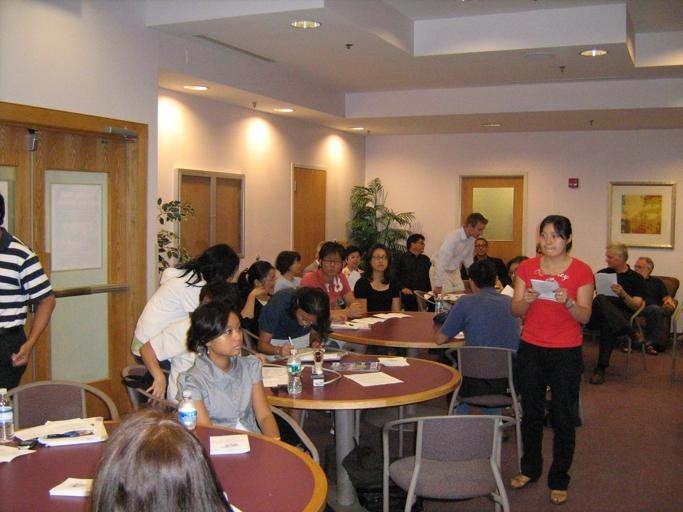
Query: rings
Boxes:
[{"xmin": 559, "ymin": 291, "xmax": 563, "ymax": 295}]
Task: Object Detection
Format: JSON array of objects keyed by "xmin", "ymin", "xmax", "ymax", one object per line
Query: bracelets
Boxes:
[{"xmin": 566, "ymin": 300, "xmax": 575, "ymax": 309}]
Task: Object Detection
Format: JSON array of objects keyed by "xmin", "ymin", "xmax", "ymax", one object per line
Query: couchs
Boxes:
[{"xmin": 654, "ymin": 276, "xmax": 680, "ymax": 352}]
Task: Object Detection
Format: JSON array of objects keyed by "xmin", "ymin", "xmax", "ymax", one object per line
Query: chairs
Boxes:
[
  {"xmin": 581, "ymin": 296, "xmax": 648, "ymax": 373},
  {"xmin": 123, "ymin": 366, "xmax": 170, "ymax": 410},
  {"xmin": 382, "ymin": 415, "xmax": 517, "ymax": 512},
  {"xmin": 413, "ymin": 290, "xmax": 436, "ymax": 311},
  {"xmin": 7, "ymin": 381, "xmax": 120, "ymax": 431},
  {"xmin": 449, "ymin": 345, "xmax": 522, "ymax": 473}
]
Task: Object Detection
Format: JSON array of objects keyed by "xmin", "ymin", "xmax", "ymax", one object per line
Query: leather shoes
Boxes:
[
  {"xmin": 631, "ymin": 330, "xmax": 641, "ymax": 348},
  {"xmin": 590, "ymin": 369, "xmax": 605, "ymax": 383}
]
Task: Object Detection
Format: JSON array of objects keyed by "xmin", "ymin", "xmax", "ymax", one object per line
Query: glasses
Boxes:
[
  {"xmin": 508, "ymin": 270, "xmax": 522, "ymax": 275},
  {"xmin": 477, "ymin": 243, "xmax": 487, "ymax": 246},
  {"xmin": 372, "ymin": 255, "xmax": 387, "ymax": 260},
  {"xmin": 414, "ymin": 240, "xmax": 424, "ymax": 244},
  {"xmin": 298, "ymin": 310, "xmax": 320, "ymax": 328},
  {"xmin": 321, "ymin": 257, "xmax": 343, "ymax": 265}
]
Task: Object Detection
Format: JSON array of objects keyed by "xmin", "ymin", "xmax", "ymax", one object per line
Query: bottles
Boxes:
[
  {"xmin": 435, "ymin": 294, "xmax": 443, "ymax": 316},
  {"xmin": 0, "ymin": 388, "xmax": 14, "ymax": 444},
  {"xmin": 178, "ymin": 390, "xmax": 196, "ymax": 437},
  {"xmin": 288, "ymin": 349, "xmax": 302, "ymax": 395}
]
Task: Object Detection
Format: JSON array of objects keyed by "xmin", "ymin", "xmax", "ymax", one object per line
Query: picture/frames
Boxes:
[{"xmin": 607, "ymin": 180, "xmax": 674, "ymax": 249}]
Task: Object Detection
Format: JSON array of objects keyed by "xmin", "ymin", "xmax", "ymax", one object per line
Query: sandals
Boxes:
[
  {"xmin": 623, "ymin": 343, "xmax": 631, "ymax": 352},
  {"xmin": 511, "ymin": 473, "xmax": 530, "ymax": 490},
  {"xmin": 647, "ymin": 342, "xmax": 656, "ymax": 354},
  {"xmin": 550, "ymin": 489, "xmax": 566, "ymax": 504}
]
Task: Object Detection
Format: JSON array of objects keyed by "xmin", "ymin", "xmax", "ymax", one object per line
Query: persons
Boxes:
[
  {"xmin": 0, "ymin": 197, "xmax": 55, "ymax": 400},
  {"xmin": 338, "ymin": 246, "xmax": 365, "ymax": 292},
  {"xmin": 180, "ymin": 304, "xmax": 281, "ymax": 442},
  {"xmin": 355, "ymin": 245, "xmax": 402, "ymax": 317},
  {"xmin": 633, "ymin": 257, "xmax": 676, "ymax": 353},
  {"xmin": 305, "ymin": 242, "xmax": 366, "ymax": 326},
  {"xmin": 470, "ymin": 237, "xmax": 511, "ymax": 296},
  {"xmin": 195, "ymin": 281, "xmax": 236, "ymax": 309},
  {"xmin": 258, "ymin": 286, "xmax": 328, "ymax": 349},
  {"xmin": 429, "ymin": 214, "xmax": 488, "ymax": 317},
  {"xmin": 436, "ymin": 258, "xmax": 523, "ymax": 422},
  {"xmin": 511, "ymin": 216, "xmax": 594, "ymax": 504},
  {"xmin": 587, "ymin": 242, "xmax": 647, "ymax": 383},
  {"xmin": 237, "ymin": 262, "xmax": 275, "ymax": 332},
  {"xmin": 400, "ymin": 234, "xmax": 434, "ymax": 312},
  {"xmin": 133, "ymin": 245, "xmax": 238, "ymax": 403},
  {"xmin": 88, "ymin": 410, "xmax": 234, "ymax": 508},
  {"xmin": 275, "ymin": 251, "xmax": 304, "ymax": 299}
]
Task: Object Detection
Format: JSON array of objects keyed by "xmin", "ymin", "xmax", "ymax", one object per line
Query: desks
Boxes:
[
  {"xmin": 443, "ymin": 289, "xmax": 473, "ymax": 307},
  {"xmin": 327, "ymin": 311, "xmax": 465, "ymax": 348},
  {"xmin": 266, "ymin": 353, "xmax": 463, "ymax": 512},
  {"xmin": 0, "ymin": 419, "xmax": 328, "ymax": 512}
]
